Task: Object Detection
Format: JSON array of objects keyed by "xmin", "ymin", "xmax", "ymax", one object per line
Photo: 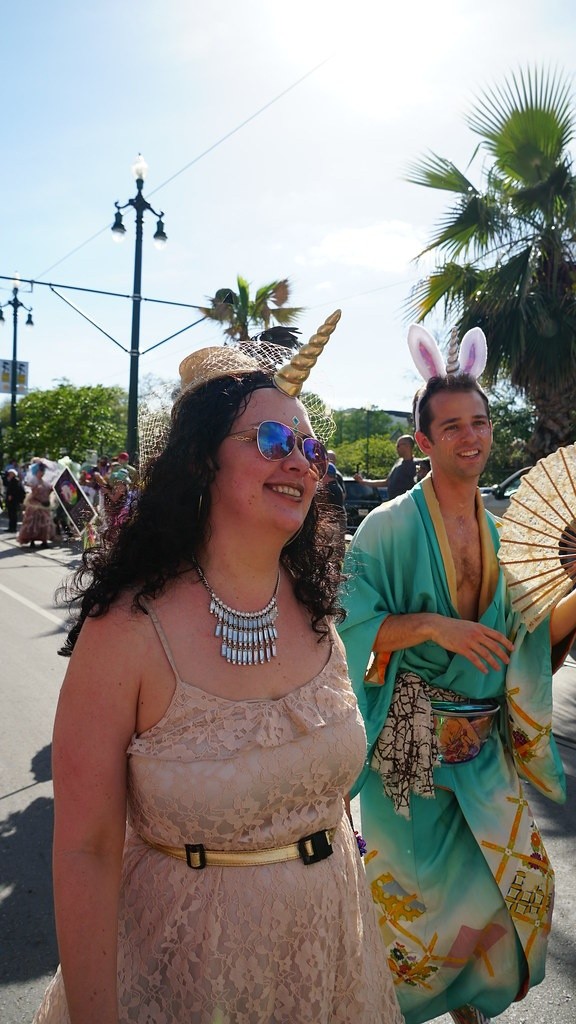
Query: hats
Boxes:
[
  {"xmin": 328, "ymin": 463, "xmax": 336, "ymax": 473},
  {"xmin": 113, "ymin": 469, "xmax": 132, "ymax": 483},
  {"xmin": 98, "ymin": 455, "xmax": 109, "ymax": 462},
  {"xmin": 119, "ymin": 452, "xmax": 129, "ymax": 460}
]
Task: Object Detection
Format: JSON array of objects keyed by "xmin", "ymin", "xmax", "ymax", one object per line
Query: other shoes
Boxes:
[
  {"xmin": 41, "ymin": 543, "xmax": 48, "ymax": 548},
  {"xmin": 4, "ymin": 529, "xmax": 16, "ymax": 533},
  {"xmin": 30, "ymin": 544, "xmax": 36, "ymax": 548},
  {"xmin": 450, "ymin": 1004, "xmax": 491, "ymax": 1024}
]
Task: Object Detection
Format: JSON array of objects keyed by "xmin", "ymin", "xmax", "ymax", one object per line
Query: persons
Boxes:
[
  {"xmin": 0, "ymin": 452, "xmax": 140, "ymax": 549},
  {"xmin": 324, "ymin": 450, "xmax": 346, "ymax": 569},
  {"xmin": 354, "ymin": 435, "xmax": 430, "ymax": 500},
  {"xmin": 31, "ymin": 310, "xmax": 403, "ymax": 1024},
  {"xmin": 336, "ymin": 323, "xmax": 576, "ymax": 1024}
]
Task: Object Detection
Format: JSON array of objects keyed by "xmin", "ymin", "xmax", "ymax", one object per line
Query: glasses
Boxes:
[
  {"xmin": 396, "ymin": 443, "xmax": 407, "ymax": 448},
  {"xmin": 37, "ymin": 468, "xmax": 45, "ymax": 472},
  {"xmin": 223, "ymin": 420, "xmax": 328, "ymax": 480}
]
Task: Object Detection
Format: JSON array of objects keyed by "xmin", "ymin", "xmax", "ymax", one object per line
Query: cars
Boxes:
[
  {"xmin": 343, "ymin": 476, "xmax": 382, "ymax": 535},
  {"xmin": 482, "ymin": 466, "xmax": 533, "ymax": 517}
]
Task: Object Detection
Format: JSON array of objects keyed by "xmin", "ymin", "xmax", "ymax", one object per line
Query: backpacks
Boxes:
[{"xmin": 19, "ymin": 483, "xmax": 26, "ymax": 504}]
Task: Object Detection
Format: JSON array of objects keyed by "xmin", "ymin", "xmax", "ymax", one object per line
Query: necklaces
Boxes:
[{"xmin": 198, "ymin": 568, "xmax": 281, "ymax": 666}]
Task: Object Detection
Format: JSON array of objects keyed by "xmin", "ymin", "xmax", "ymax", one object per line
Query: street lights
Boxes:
[
  {"xmin": 110, "ymin": 151, "xmax": 168, "ymax": 474},
  {"xmin": 0, "ymin": 270, "xmax": 35, "ymax": 428}
]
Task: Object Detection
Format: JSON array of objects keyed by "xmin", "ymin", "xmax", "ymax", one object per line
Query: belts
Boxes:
[{"xmin": 139, "ymin": 828, "xmax": 339, "ymax": 869}]
